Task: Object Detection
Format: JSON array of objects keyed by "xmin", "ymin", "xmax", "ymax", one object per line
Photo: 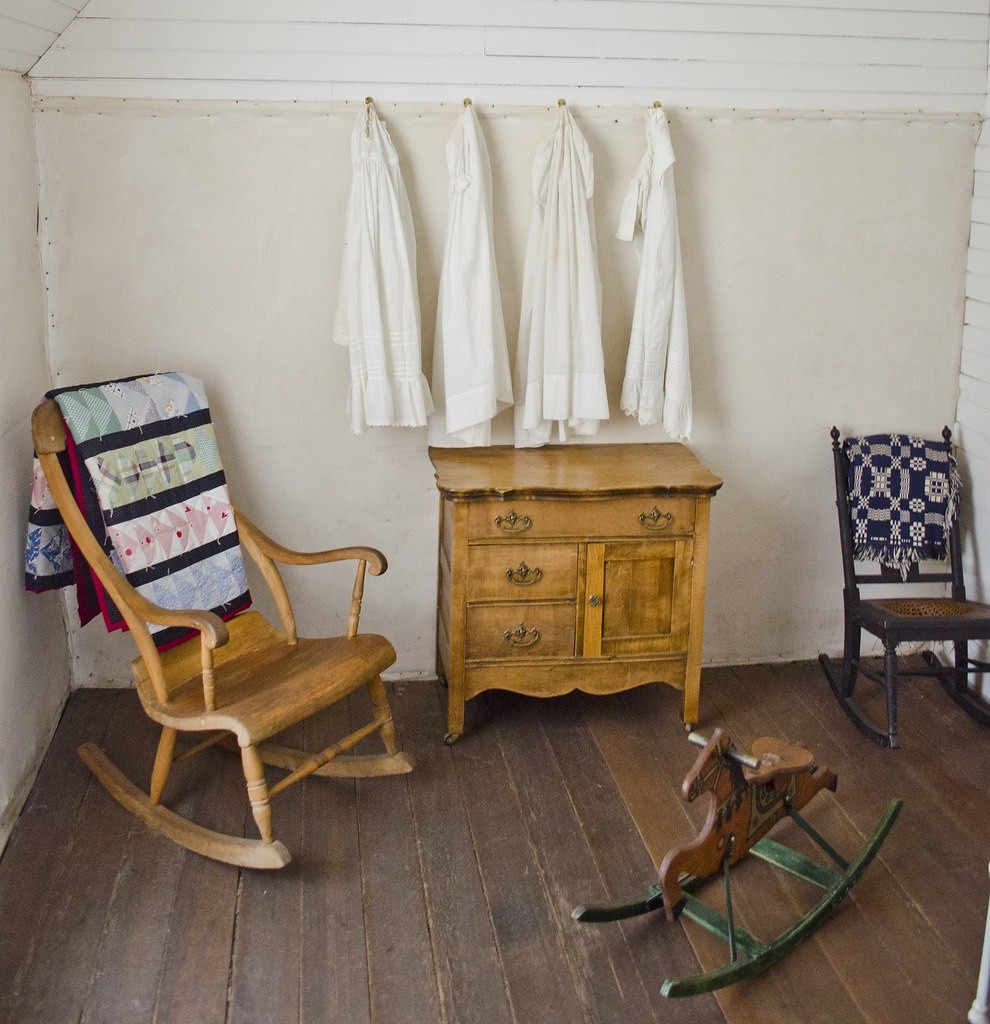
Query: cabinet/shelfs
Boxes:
[{"xmin": 429, "ymin": 443, "xmax": 722, "ymax": 746}]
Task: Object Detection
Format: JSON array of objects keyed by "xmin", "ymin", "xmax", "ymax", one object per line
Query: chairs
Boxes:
[
  {"xmin": 32, "ymin": 398, "xmax": 417, "ymax": 869},
  {"xmin": 817, "ymin": 426, "xmax": 990, "ymax": 751}
]
